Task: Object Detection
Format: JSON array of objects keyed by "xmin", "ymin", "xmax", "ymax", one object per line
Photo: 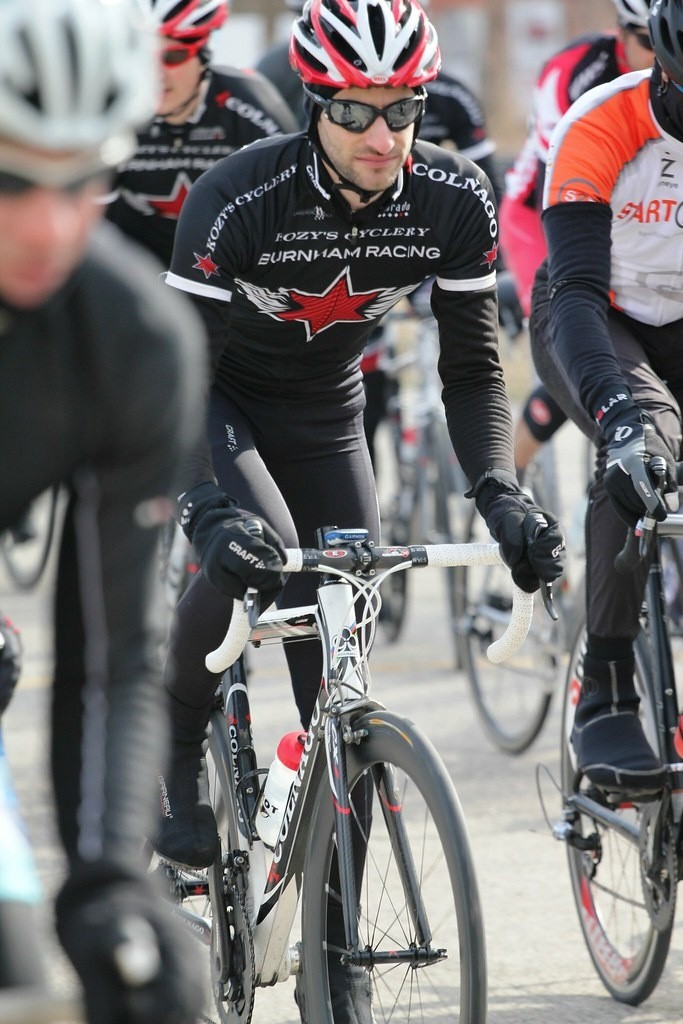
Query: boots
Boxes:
[
  {"xmin": 148, "ymin": 683, "xmax": 218, "ymax": 871},
  {"xmin": 568, "ymin": 654, "xmax": 670, "ymax": 793}
]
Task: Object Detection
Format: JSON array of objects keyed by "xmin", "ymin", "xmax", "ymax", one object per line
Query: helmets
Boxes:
[
  {"xmin": 0, "ymin": 0, "xmax": 163, "ymax": 193},
  {"xmin": 149, "ymin": 1, "xmax": 226, "ymax": 41},
  {"xmin": 287, "ymin": 0, "xmax": 443, "ymax": 88},
  {"xmin": 613, "ymin": 0, "xmax": 683, "ymax": 87}
]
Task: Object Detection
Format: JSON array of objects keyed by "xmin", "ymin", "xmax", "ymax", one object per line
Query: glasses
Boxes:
[
  {"xmin": 300, "ymin": 81, "xmax": 429, "ymax": 133},
  {"xmin": 629, "ymin": 28, "xmax": 653, "ymax": 52},
  {"xmin": 160, "ymin": 39, "xmax": 208, "ymax": 69}
]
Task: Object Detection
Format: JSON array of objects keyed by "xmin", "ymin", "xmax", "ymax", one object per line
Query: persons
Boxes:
[
  {"xmin": 105, "ymin": 1, "xmax": 299, "ymax": 270},
  {"xmin": 0, "ymin": 0, "xmax": 213, "ymax": 1024},
  {"xmin": 529, "ymin": 0, "xmax": 683, "ymax": 796},
  {"xmin": 250, "ymin": 0, "xmax": 656, "ymax": 491},
  {"xmin": 142, "ymin": 0, "xmax": 564, "ymax": 857}
]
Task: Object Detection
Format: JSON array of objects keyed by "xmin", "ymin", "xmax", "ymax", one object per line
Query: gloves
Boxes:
[
  {"xmin": 52, "ymin": 859, "xmax": 207, "ymax": 1023},
  {"xmin": 603, "ymin": 408, "xmax": 678, "ymax": 531},
  {"xmin": 460, "ymin": 467, "xmax": 568, "ymax": 594},
  {"xmin": 170, "ymin": 481, "xmax": 291, "ymax": 606}
]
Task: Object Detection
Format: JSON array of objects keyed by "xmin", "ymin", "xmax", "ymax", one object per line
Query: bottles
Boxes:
[{"xmin": 255, "ymin": 732, "xmax": 308, "ymax": 848}]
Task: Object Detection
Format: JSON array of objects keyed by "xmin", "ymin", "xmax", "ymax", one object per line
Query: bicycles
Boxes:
[
  {"xmin": 366, "ymin": 272, "xmax": 523, "ymax": 668},
  {"xmin": 536, "ymin": 455, "xmax": 683, "ymax": 1005},
  {"xmin": 453, "ymin": 464, "xmax": 683, "ymax": 749},
  {"xmin": 143, "ymin": 505, "xmax": 558, "ymax": 1024}
]
